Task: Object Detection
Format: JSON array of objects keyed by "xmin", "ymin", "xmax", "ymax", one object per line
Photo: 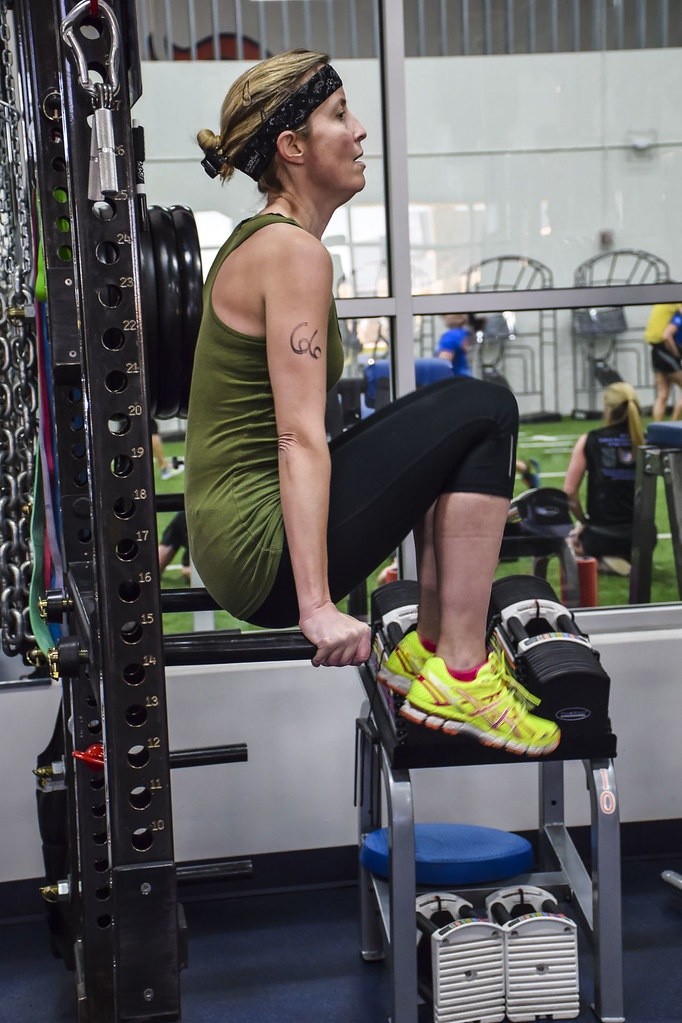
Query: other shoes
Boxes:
[{"xmin": 598, "ymin": 556, "xmax": 631, "ymax": 576}]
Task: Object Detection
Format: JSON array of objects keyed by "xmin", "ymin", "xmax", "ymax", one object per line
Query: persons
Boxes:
[
  {"xmin": 434, "ymin": 312, "xmax": 539, "ymax": 490},
  {"xmin": 184, "ymin": 46, "xmax": 562, "ymax": 757},
  {"xmin": 564, "ymin": 382, "xmax": 657, "ymax": 575},
  {"xmin": 644, "ymin": 302, "xmax": 682, "ymax": 424}
]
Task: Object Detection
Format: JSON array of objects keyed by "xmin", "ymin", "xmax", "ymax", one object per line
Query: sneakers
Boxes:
[
  {"xmin": 399, "ymin": 650, "xmax": 560, "ymax": 755},
  {"xmin": 377, "ymin": 631, "xmax": 436, "ymax": 695}
]
